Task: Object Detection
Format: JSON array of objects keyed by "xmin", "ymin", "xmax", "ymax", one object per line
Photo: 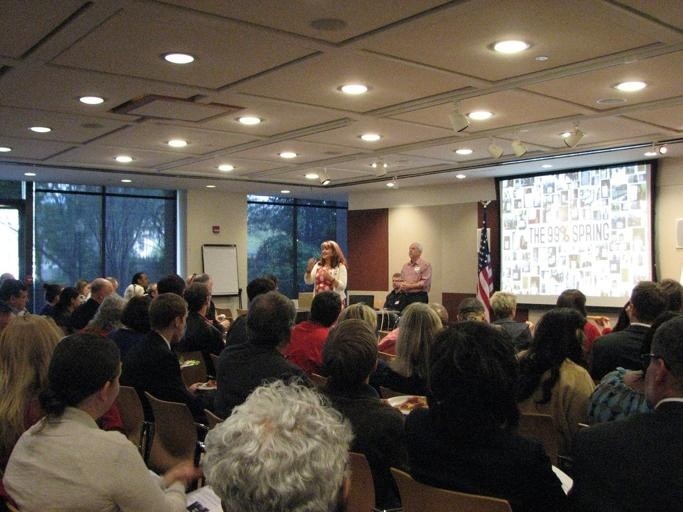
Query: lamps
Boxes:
[
  {"xmin": 659, "ymin": 146, "xmax": 668, "ymax": 155},
  {"xmin": 375, "ymin": 156, "xmax": 387, "ymax": 177},
  {"xmin": 511, "ymin": 128, "xmax": 528, "ymax": 158},
  {"xmin": 319, "ymin": 168, "xmax": 331, "ymax": 185},
  {"xmin": 487, "ymin": 135, "xmax": 504, "ymax": 160},
  {"xmin": 563, "ymin": 124, "xmax": 584, "ymax": 148},
  {"xmin": 448, "ymin": 100, "xmax": 469, "ymax": 132}
]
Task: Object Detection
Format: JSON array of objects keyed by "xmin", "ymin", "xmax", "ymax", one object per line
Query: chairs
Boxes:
[
  {"xmin": 215, "ymin": 308, "xmax": 234, "ymax": 340},
  {"xmin": 377, "ymin": 350, "xmax": 397, "ymax": 363},
  {"xmin": 236, "ymin": 309, "xmax": 249, "ymax": 317},
  {"xmin": 311, "ymin": 372, "xmax": 328, "ymax": 388},
  {"xmin": 379, "ymin": 386, "xmax": 406, "ymax": 399},
  {"xmin": 346, "ymin": 452, "xmax": 375, "ymax": 512},
  {"xmin": 113, "ymin": 350, "xmax": 224, "ymax": 474},
  {"xmin": 390, "ymin": 467, "xmax": 512, "ymax": 512},
  {"xmin": 516, "ymin": 413, "xmax": 558, "ymax": 466}
]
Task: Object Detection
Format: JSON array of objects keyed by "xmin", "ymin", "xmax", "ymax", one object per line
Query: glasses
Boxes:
[{"xmin": 639, "ymin": 352, "xmax": 667, "ymax": 370}]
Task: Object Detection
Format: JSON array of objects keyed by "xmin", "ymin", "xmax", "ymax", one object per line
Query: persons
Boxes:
[
  {"xmin": 304, "ymin": 241, "xmax": 346, "ymax": 308},
  {"xmin": 1, "ymin": 272, "xmax": 682, "ymax": 512},
  {"xmin": 402, "ymin": 241, "xmax": 431, "ymax": 304}
]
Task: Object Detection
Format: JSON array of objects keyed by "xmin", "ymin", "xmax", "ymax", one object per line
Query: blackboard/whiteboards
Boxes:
[{"xmin": 201, "ymin": 244, "xmax": 240, "ymax": 297}]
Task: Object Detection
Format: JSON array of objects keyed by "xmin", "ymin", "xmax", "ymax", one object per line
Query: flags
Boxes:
[{"xmin": 471, "ymin": 220, "xmax": 493, "ymax": 325}]
